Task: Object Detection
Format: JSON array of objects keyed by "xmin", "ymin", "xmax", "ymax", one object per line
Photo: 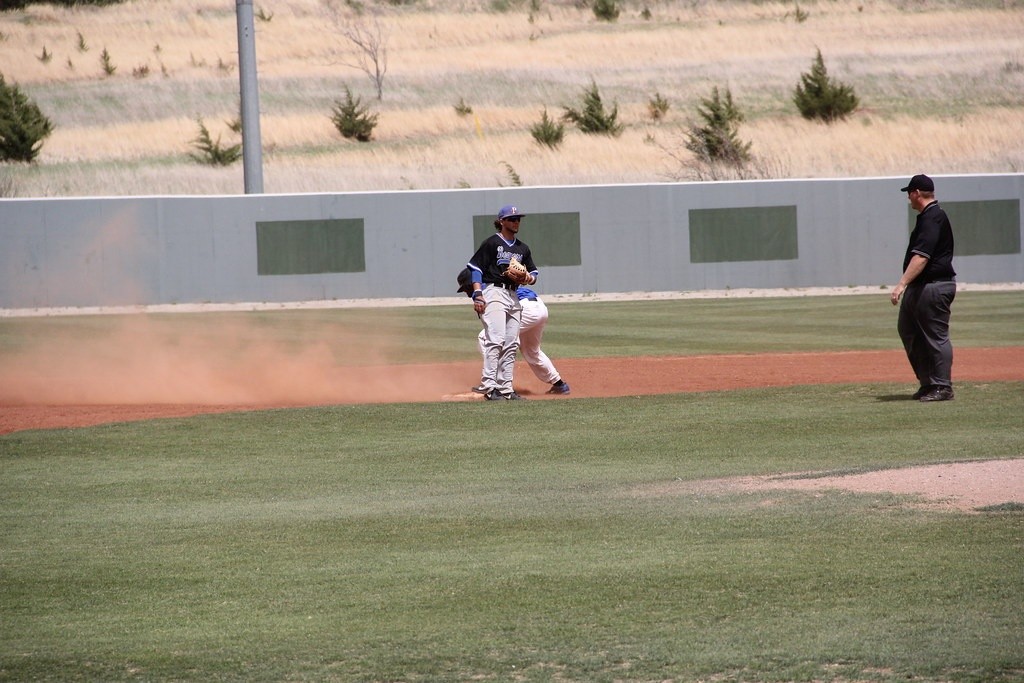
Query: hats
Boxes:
[
  {"xmin": 901, "ymin": 174, "xmax": 934, "ymax": 192},
  {"xmin": 456, "ymin": 268, "xmax": 473, "ymax": 293},
  {"xmin": 498, "ymin": 205, "xmax": 525, "ymax": 220}
]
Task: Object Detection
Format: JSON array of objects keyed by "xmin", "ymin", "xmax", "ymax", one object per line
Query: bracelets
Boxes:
[{"xmin": 474, "ymin": 289, "xmax": 482, "ymax": 296}]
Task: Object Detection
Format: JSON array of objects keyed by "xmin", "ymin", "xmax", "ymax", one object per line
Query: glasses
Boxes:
[{"xmin": 505, "ymin": 217, "xmax": 520, "ymax": 222}]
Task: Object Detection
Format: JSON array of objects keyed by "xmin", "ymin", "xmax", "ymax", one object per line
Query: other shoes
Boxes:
[{"xmin": 912, "ymin": 385, "xmax": 954, "ymax": 402}]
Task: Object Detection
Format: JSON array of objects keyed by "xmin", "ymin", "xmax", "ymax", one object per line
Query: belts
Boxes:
[
  {"xmin": 494, "ymin": 283, "xmax": 515, "ymax": 291},
  {"xmin": 528, "ymin": 298, "xmax": 537, "ymax": 301}
]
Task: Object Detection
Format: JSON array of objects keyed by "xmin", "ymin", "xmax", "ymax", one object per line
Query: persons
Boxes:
[
  {"xmin": 457, "ymin": 268, "xmax": 571, "ymax": 395},
  {"xmin": 467, "ymin": 205, "xmax": 538, "ymax": 400},
  {"xmin": 891, "ymin": 175, "xmax": 958, "ymax": 400}
]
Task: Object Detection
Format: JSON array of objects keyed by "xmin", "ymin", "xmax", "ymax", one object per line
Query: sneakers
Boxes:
[
  {"xmin": 484, "ymin": 389, "xmax": 521, "ymax": 401},
  {"xmin": 472, "ymin": 385, "xmax": 486, "ymax": 393},
  {"xmin": 544, "ymin": 382, "xmax": 569, "ymax": 395}
]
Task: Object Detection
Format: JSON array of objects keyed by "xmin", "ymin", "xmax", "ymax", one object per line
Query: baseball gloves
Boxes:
[{"xmin": 503, "ymin": 256, "xmax": 531, "ymax": 286}]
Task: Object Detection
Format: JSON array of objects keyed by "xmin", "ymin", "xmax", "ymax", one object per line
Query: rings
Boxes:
[{"xmin": 893, "ymin": 297, "xmax": 895, "ymax": 299}]
